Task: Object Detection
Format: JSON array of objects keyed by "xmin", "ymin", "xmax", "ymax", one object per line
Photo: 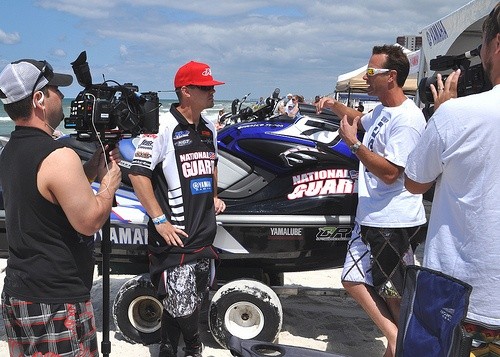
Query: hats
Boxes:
[
  {"xmin": 0, "ymin": 59, "xmax": 73, "ymax": 104},
  {"xmin": 175, "ymin": 61, "xmax": 226, "ymax": 88}
]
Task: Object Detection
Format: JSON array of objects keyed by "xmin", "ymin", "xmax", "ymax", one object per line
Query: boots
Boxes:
[
  {"xmin": 177, "ymin": 308, "xmax": 204, "ymax": 357},
  {"xmin": 159, "ymin": 309, "xmax": 183, "ymax": 357}
]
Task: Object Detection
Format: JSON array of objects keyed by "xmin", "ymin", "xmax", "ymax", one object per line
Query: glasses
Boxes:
[
  {"xmin": 197, "ymin": 86, "xmax": 214, "ymax": 91},
  {"xmin": 366, "ymin": 68, "xmax": 398, "ymax": 77},
  {"xmin": 32, "ymin": 60, "xmax": 52, "ymax": 109},
  {"xmin": 292, "ymin": 99, "xmax": 295, "ymax": 101}
]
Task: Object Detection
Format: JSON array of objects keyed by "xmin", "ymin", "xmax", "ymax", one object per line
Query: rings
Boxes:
[{"xmin": 438, "ymin": 87, "xmax": 444, "ymax": 90}]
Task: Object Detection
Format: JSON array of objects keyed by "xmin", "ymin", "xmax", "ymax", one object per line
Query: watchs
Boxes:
[{"xmin": 350, "ymin": 141, "xmax": 361, "ymax": 154}]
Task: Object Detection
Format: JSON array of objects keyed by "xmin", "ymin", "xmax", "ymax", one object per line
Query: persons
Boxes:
[
  {"xmin": 402, "ymin": 1, "xmax": 500, "ymax": 342},
  {"xmin": 312, "ymin": 95, "xmax": 320, "ymax": 107},
  {"xmin": 272, "ymin": 93, "xmax": 306, "ymax": 119},
  {"xmin": 216, "ymin": 110, "xmax": 226, "ymax": 131},
  {"xmin": 0, "ymin": 58, "xmax": 122, "ymax": 357},
  {"xmin": 313, "ymin": 45, "xmax": 426, "ymax": 357},
  {"xmin": 357, "ymin": 102, "xmax": 364, "ymax": 114},
  {"xmin": 128, "ymin": 61, "xmax": 226, "ymax": 357}
]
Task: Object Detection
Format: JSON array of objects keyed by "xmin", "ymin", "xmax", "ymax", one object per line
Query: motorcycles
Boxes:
[{"xmin": 2, "ymin": 87, "xmax": 433, "ymax": 286}]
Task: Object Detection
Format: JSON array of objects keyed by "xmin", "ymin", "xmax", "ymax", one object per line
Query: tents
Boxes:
[
  {"xmin": 333, "ymin": 43, "xmax": 421, "ymax": 111},
  {"xmin": 415, "ymin": 0, "xmax": 500, "ymax": 107}
]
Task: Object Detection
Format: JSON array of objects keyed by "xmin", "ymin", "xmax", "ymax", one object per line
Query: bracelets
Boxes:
[{"xmin": 152, "ymin": 214, "xmax": 167, "ymax": 224}]
